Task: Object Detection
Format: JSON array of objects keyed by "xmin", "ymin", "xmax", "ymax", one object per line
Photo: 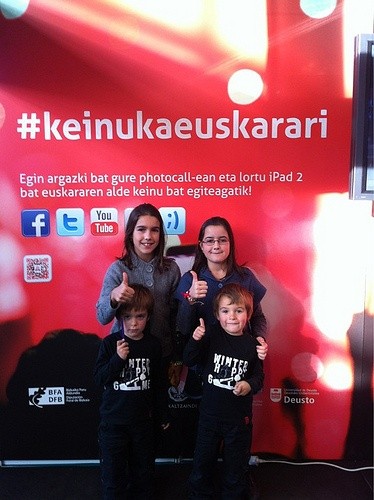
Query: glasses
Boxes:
[{"xmin": 202, "ymin": 238, "xmax": 230, "ymax": 245}]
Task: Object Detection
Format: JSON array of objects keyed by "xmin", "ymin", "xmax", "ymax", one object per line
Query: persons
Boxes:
[
  {"xmin": 93, "ymin": 284, "xmax": 171, "ymax": 500},
  {"xmin": 175, "ymin": 216, "xmax": 269, "ymax": 450},
  {"xmin": 182, "ymin": 282, "xmax": 265, "ymax": 500},
  {"xmin": 96, "ymin": 204, "xmax": 181, "ymax": 447}
]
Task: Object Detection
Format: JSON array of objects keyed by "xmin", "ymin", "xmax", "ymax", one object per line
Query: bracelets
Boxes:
[{"xmin": 186, "ymin": 289, "xmax": 195, "ymax": 303}]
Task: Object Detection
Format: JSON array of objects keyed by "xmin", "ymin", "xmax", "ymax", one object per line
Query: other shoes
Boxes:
[{"xmin": 243, "ymin": 472, "xmax": 261, "ymax": 500}]
